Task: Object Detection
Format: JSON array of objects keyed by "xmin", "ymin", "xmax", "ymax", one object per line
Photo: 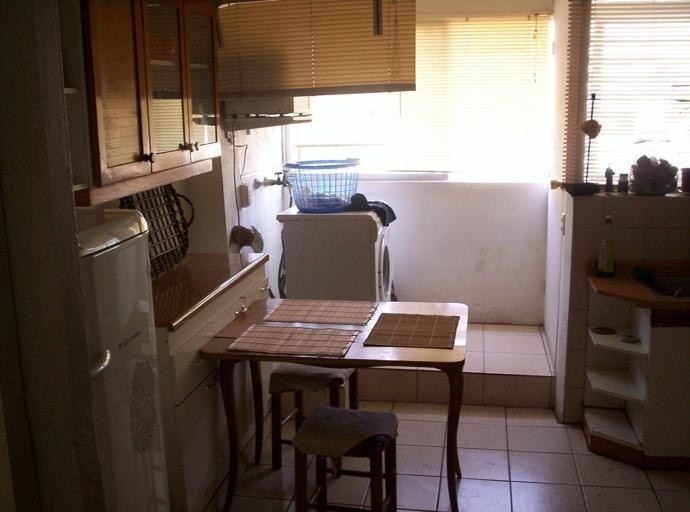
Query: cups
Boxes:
[{"xmin": 680, "ymin": 168, "xmax": 690, "ymax": 191}]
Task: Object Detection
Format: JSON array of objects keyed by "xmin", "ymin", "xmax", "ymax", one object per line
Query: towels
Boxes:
[{"xmin": 346, "ymin": 193, "xmax": 398, "ymax": 228}]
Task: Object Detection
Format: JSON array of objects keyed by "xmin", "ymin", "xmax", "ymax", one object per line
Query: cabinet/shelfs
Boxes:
[
  {"xmin": 554, "ymin": 192, "xmax": 690, "ymax": 427},
  {"xmin": 55, "ymin": 3, "xmax": 95, "ymax": 194},
  {"xmin": 137, "ymin": 2, "xmax": 223, "ymax": 189},
  {"xmin": 80, "ymin": 1, "xmax": 153, "ymax": 206},
  {"xmin": 581, "ymin": 259, "xmax": 689, "ymax": 468},
  {"xmin": 157, "ymin": 251, "xmax": 271, "ymax": 511}
]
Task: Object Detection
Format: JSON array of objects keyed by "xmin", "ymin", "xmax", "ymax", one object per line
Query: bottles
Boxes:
[
  {"xmin": 598, "ymin": 214, "xmax": 614, "ymax": 273},
  {"xmin": 618, "ymin": 173, "xmax": 629, "ymax": 192}
]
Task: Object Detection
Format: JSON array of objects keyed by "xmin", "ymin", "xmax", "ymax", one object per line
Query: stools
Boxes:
[
  {"xmin": 293, "ymin": 407, "xmax": 398, "ymax": 511},
  {"xmin": 269, "ymin": 361, "xmax": 359, "ymax": 478}
]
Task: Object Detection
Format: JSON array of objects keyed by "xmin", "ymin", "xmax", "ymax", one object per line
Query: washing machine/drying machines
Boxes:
[{"xmin": 275, "ymin": 204, "xmax": 392, "ymax": 302}]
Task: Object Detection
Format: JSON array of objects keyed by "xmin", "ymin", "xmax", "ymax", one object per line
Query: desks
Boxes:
[{"xmin": 199, "ymin": 300, "xmax": 471, "ymax": 512}]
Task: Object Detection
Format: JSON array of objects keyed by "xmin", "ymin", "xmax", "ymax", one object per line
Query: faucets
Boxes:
[
  {"xmin": 254, "ymin": 171, "xmax": 287, "ymax": 189},
  {"xmin": 264, "ymin": 169, "xmax": 292, "ymax": 187}
]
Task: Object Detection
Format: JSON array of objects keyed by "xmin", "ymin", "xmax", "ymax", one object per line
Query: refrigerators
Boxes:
[{"xmin": 73, "ymin": 201, "xmax": 173, "ymax": 512}]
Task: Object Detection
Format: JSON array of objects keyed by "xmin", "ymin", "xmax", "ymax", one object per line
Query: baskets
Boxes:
[{"xmin": 283, "ymin": 159, "xmax": 360, "ymax": 213}]
